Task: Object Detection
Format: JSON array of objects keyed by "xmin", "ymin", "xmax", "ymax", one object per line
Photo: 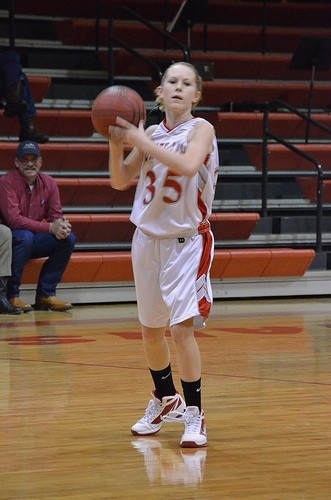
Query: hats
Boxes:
[{"xmin": 16, "ymin": 141, "xmax": 40, "ymax": 159}]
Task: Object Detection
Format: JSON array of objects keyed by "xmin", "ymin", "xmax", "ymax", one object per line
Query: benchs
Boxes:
[{"xmin": 0, "ymin": 1, "xmax": 331, "ymax": 285}]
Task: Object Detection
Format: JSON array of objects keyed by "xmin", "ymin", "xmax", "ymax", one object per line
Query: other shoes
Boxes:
[
  {"xmin": 0, "ymin": 295, "xmax": 21, "ymax": 314},
  {"xmin": 19, "ymin": 132, "xmax": 49, "ymax": 144}
]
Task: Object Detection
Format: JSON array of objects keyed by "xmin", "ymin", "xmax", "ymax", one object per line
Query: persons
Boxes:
[
  {"xmin": 0, "ymin": 140, "xmax": 77, "ymax": 311},
  {"xmin": 107, "ymin": 63, "xmax": 219, "ymax": 449},
  {"xmin": 0, "ymin": 45, "xmax": 49, "ymax": 143},
  {"xmin": 0, "ymin": 223, "xmax": 22, "ymax": 314}
]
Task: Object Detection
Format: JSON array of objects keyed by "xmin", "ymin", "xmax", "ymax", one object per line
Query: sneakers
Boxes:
[
  {"xmin": 131, "ymin": 390, "xmax": 186, "ymax": 436},
  {"xmin": 162, "ymin": 406, "xmax": 207, "ymax": 447}
]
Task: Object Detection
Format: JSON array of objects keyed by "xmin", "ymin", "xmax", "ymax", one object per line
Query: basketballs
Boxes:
[{"xmin": 91, "ymin": 85, "xmax": 146, "ymax": 142}]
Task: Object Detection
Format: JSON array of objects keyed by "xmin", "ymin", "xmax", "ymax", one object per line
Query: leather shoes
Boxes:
[
  {"xmin": 35, "ymin": 295, "xmax": 72, "ymax": 311},
  {"xmin": 8, "ymin": 297, "xmax": 32, "ymax": 311}
]
